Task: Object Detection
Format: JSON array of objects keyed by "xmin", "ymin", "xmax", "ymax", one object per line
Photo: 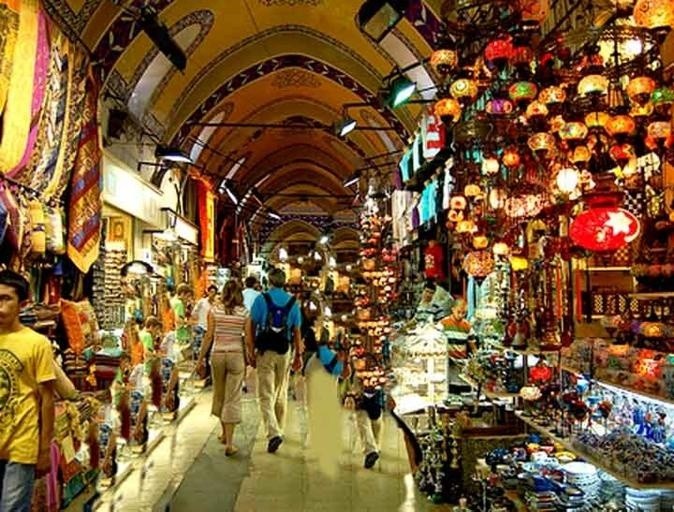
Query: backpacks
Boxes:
[{"xmin": 256, "ymin": 292, "xmax": 296, "ymax": 354}]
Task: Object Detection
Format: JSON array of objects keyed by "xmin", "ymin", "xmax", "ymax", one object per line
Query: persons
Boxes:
[
  {"xmin": 437, "ymin": 298, "xmax": 479, "ymax": 367},
  {"xmin": 0, "ymin": 270, "xmax": 57, "ymax": 512},
  {"xmin": 397, "ymin": 282, "xmax": 444, "ymax": 333}
]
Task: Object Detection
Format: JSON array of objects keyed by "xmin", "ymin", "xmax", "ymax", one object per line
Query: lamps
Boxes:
[{"xmin": 339, "ymin": 58, "xmax": 440, "ymax": 187}]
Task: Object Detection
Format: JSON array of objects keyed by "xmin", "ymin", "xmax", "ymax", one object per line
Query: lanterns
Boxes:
[{"xmin": 640, "ymin": 322, "xmax": 667, "ymax": 337}]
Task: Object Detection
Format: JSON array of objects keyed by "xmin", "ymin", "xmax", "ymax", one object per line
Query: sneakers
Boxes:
[
  {"xmin": 268, "ymin": 437, "xmax": 282, "ymax": 452},
  {"xmin": 365, "ymin": 452, "xmax": 378, "ymax": 468},
  {"xmin": 217, "ymin": 432, "xmax": 238, "ymax": 456}
]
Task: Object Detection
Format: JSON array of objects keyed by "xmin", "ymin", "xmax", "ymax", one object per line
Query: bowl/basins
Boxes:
[{"xmin": 561, "ymin": 458, "xmax": 673, "ymax": 512}]
